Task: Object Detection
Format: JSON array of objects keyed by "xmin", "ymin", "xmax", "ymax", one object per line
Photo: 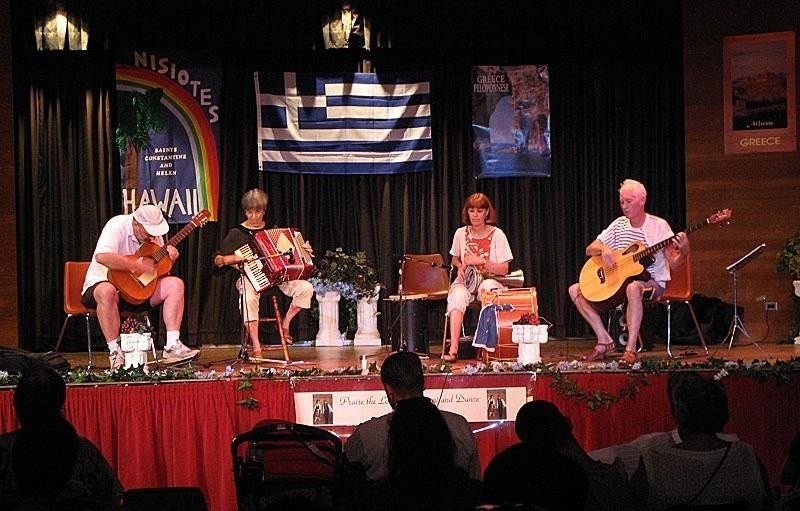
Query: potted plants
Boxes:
[
  {"xmin": 774, "ymin": 235, "xmax": 800, "ymax": 298},
  {"xmin": 305, "ymin": 247, "xmax": 387, "ymax": 347}
]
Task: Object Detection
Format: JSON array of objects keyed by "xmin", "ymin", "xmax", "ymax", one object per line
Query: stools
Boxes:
[{"xmin": 241, "ymin": 287, "xmax": 292, "ymax": 364}]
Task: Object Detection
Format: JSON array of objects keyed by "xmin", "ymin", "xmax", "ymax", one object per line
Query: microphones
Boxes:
[
  {"xmin": 438, "ymin": 264, "xmax": 458, "ymax": 273},
  {"xmin": 287, "ymin": 248, "xmax": 295, "ymax": 265}
]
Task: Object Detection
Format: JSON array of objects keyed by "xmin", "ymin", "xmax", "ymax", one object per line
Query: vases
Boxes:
[
  {"xmin": 118, "ymin": 333, "xmax": 154, "ymax": 372},
  {"xmin": 513, "ymin": 323, "xmax": 548, "ymax": 365}
]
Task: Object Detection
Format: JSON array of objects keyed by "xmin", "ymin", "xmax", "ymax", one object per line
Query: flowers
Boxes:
[
  {"xmin": 121, "ymin": 317, "xmax": 146, "ymax": 333},
  {"xmin": 517, "ymin": 312, "xmax": 538, "ymax": 325}
]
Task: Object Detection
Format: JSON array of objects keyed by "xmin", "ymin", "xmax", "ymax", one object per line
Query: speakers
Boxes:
[{"xmin": 610, "ymin": 303, "xmax": 659, "ymax": 352}]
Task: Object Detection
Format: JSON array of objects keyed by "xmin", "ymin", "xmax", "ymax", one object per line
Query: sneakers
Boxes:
[
  {"xmin": 162, "ymin": 338, "xmax": 201, "ymax": 368},
  {"xmin": 109, "ymin": 345, "xmax": 125, "ymax": 371}
]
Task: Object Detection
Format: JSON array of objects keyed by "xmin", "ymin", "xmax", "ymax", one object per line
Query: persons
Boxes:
[
  {"xmin": 567, "ymin": 179, "xmax": 690, "ymax": 369},
  {"xmin": 313, "ymin": 399, "xmax": 321, "ymax": 424},
  {"xmin": 215, "ymin": 188, "xmax": 316, "ymax": 365},
  {"xmin": 81, "ymin": 205, "xmax": 201, "ymax": 374},
  {"xmin": 0, "ymin": 366, "xmax": 130, "ymax": 511},
  {"xmin": 482, "ymin": 400, "xmax": 629, "ymax": 511},
  {"xmin": 0, "ymin": 414, "xmax": 113, "ymax": 511},
  {"xmin": 343, "ymin": 352, "xmax": 480, "ymax": 511},
  {"xmin": 628, "ymin": 369, "xmax": 773, "ymax": 511},
  {"xmin": 323, "ymin": 400, "xmax": 333, "ymax": 424},
  {"xmin": 443, "ymin": 192, "xmax": 514, "ymax": 363}
]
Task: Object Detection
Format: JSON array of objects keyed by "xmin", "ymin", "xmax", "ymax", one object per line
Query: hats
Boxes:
[{"xmin": 134, "ymin": 204, "xmax": 169, "ymax": 237}]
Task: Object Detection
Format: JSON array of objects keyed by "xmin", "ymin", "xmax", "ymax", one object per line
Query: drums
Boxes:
[{"xmin": 464, "ymin": 265, "xmax": 525, "ymax": 294}]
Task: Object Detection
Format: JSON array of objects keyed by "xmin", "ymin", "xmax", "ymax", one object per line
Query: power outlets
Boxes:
[{"xmin": 765, "ymin": 302, "xmax": 778, "ymax": 310}]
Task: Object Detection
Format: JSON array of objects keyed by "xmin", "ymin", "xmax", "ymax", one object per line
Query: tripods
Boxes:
[
  {"xmin": 358, "ymin": 261, "xmax": 446, "ymax": 363},
  {"xmin": 203, "ymin": 264, "xmax": 305, "ymax": 370},
  {"xmin": 712, "ymin": 270, "xmax": 773, "ymax": 359}
]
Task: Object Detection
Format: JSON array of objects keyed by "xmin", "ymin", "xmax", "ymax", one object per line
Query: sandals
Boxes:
[
  {"xmin": 581, "ymin": 341, "xmax": 616, "ymax": 361},
  {"xmin": 444, "ymin": 352, "xmax": 459, "ymax": 363},
  {"xmin": 250, "ymin": 349, "xmax": 263, "ymax": 365},
  {"xmin": 274, "ymin": 320, "xmax": 295, "ymax": 346},
  {"xmin": 619, "ymin": 351, "xmax": 642, "ymax": 365}
]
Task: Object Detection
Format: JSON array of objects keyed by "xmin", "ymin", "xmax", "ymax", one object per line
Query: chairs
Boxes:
[
  {"xmin": 440, "ymin": 265, "xmax": 509, "ymax": 361},
  {"xmin": 55, "ymin": 261, "xmax": 159, "ymax": 371},
  {"xmin": 608, "ymin": 237, "xmax": 709, "ymax": 358},
  {"xmin": 123, "ymin": 486, "xmax": 207, "ymax": 510},
  {"xmin": 231, "ymin": 419, "xmax": 361, "ymax": 511},
  {"xmin": 384, "ymin": 253, "xmax": 451, "ymax": 354}
]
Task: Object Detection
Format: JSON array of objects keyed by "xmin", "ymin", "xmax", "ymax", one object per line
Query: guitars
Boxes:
[
  {"xmin": 580, "ymin": 207, "xmax": 734, "ymax": 309},
  {"xmin": 107, "ymin": 209, "xmax": 211, "ymax": 305}
]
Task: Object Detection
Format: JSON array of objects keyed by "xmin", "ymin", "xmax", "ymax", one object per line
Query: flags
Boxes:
[{"xmin": 254, "ymin": 71, "xmax": 433, "ymax": 175}]
